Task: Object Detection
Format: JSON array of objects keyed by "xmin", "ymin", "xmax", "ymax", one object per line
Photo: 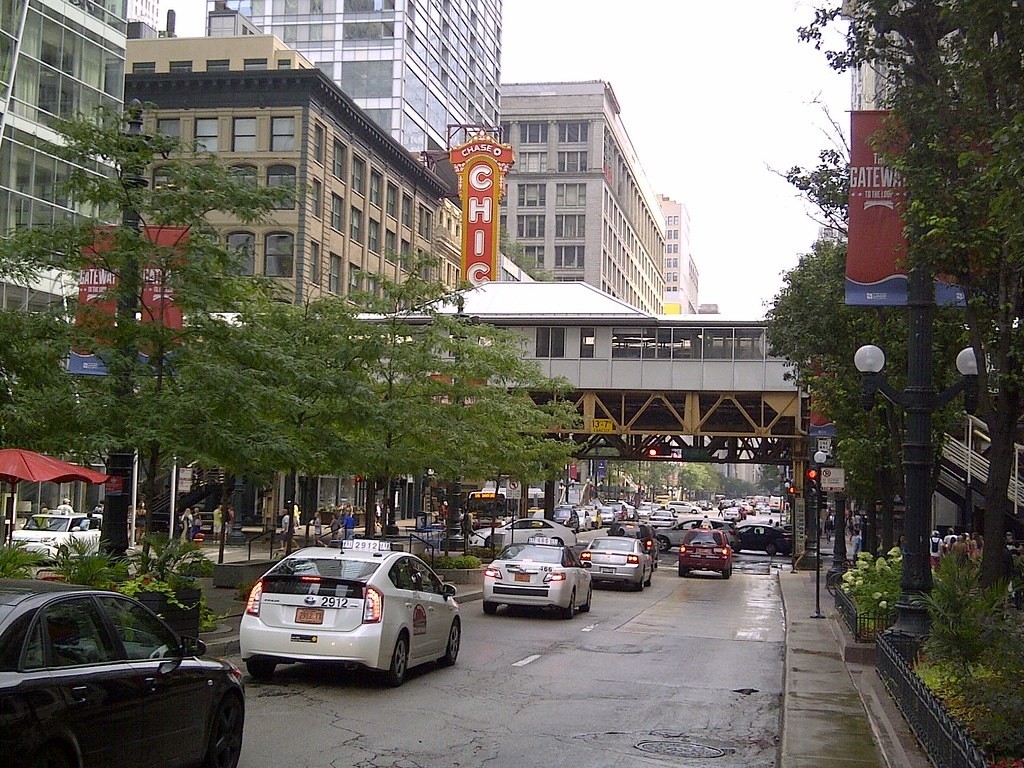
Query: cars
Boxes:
[
  {"xmin": 469, "ymin": 518, "xmax": 578, "ymax": 548},
  {"xmin": 733, "ymin": 524, "xmax": 793, "ymax": 557},
  {"xmin": 484, "ymin": 538, "xmax": 593, "ymax": 617},
  {"xmin": 427, "ymin": 537, "xmax": 467, "ymax": 557},
  {"xmin": 677, "ymin": 528, "xmax": 734, "ymax": 578},
  {"xmin": 238, "ymin": 539, "xmax": 462, "ymax": 685},
  {"xmin": 0, "ymin": 586, "xmax": 247, "ymax": 768},
  {"xmin": 579, "ymin": 537, "xmax": 655, "ymax": 591},
  {"xmin": 1, "ymin": 513, "xmax": 104, "ymax": 563},
  {"xmin": 524, "ymin": 494, "xmax": 786, "ymax": 530}
]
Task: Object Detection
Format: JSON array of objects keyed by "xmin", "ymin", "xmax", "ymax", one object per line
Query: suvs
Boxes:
[{"xmin": 655, "ymin": 518, "xmax": 742, "ymax": 552}]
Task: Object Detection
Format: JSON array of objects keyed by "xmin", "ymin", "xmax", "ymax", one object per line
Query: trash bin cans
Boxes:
[
  {"xmin": 392, "ymin": 543, "xmax": 404, "ymax": 552},
  {"xmin": 328, "ymin": 540, "xmax": 342, "ymax": 548},
  {"xmin": 380, "ymin": 524, "xmax": 400, "ymax": 535},
  {"xmin": 416, "ymin": 511, "xmax": 432, "ymax": 532}
]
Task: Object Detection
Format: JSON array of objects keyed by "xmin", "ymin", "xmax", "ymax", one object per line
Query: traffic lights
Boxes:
[
  {"xmin": 355, "ymin": 477, "xmax": 364, "ymax": 482},
  {"xmin": 788, "ymin": 485, "xmax": 794, "ymax": 495},
  {"xmin": 819, "ymin": 489, "xmax": 828, "ymax": 511},
  {"xmin": 401, "ymin": 479, "xmax": 406, "ymax": 486},
  {"xmin": 807, "ymin": 468, "xmax": 819, "ymax": 498}
]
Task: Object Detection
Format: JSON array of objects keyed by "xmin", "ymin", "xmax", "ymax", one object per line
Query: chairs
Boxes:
[{"xmin": 46, "ymin": 618, "xmax": 94, "ymax": 663}]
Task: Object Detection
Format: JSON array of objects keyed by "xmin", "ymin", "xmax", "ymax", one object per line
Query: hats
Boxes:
[
  {"xmin": 62, "ymin": 498, "xmax": 71, "ymax": 504},
  {"xmin": 1006, "ymin": 531, "xmax": 1012, "ymax": 536}
]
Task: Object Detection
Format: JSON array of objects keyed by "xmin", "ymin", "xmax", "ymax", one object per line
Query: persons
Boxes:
[
  {"xmin": 439, "ymin": 501, "xmax": 448, "ymax": 520},
  {"xmin": 853, "ymin": 530, "xmax": 862, "ymax": 560},
  {"xmin": 325, "ymin": 513, "xmax": 341, "ymax": 540},
  {"xmin": 190, "ymin": 508, "xmax": 201, "ymax": 542},
  {"xmin": 127, "ymin": 500, "xmax": 146, "ymax": 544},
  {"xmin": 459, "ymin": 509, "xmax": 473, "ymax": 533},
  {"xmin": 57, "ymin": 498, "xmax": 74, "ymax": 514},
  {"xmin": 224, "ymin": 505, "xmax": 235, "ymax": 544},
  {"xmin": 213, "ymin": 505, "xmax": 222, "ymax": 543},
  {"xmin": 374, "ymin": 500, "xmax": 384, "ymax": 533},
  {"xmin": 277, "ymin": 500, "xmax": 301, "ymax": 551},
  {"xmin": 718, "ymin": 502, "xmax": 724, "ymax": 517},
  {"xmin": 700, "ymin": 515, "xmax": 713, "ymax": 529},
  {"xmin": 92, "ymin": 500, "xmax": 105, "ymax": 514},
  {"xmin": 342, "ymin": 515, "xmax": 355, "ymax": 539},
  {"xmin": 309, "ymin": 512, "xmax": 328, "ymax": 548},
  {"xmin": 896, "ymin": 534, "xmax": 905, "ymax": 553},
  {"xmin": 824, "ymin": 508, "xmax": 861, "ymax": 544},
  {"xmin": 181, "ymin": 508, "xmax": 192, "ymax": 541},
  {"xmin": 930, "ymin": 528, "xmax": 1023, "ymax": 571}
]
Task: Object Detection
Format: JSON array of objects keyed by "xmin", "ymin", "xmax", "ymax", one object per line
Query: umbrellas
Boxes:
[{"xmin": 0, "ymin": 448, "xmax": 110, "ymax": 548}]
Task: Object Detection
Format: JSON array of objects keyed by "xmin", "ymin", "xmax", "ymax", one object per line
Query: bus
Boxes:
[{"xmin": 465, "ymin": 484, "xmax": 547, "ymax": 530}]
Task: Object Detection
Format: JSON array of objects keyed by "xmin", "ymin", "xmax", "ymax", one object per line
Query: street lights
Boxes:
[
  {"xmin": 81, "ymin": 100, "xmax": 171, "ymax": 573},
  {"xmin": 811, "ymin": 451, "xmax": 828, "ymax": 619},
  {"xmin": 428, "ymin": 468, "xmax": 434, "ymax": 513},
  {"xmin": 848, "ymin": 58, "xmax": 992, "ymax": 654}
]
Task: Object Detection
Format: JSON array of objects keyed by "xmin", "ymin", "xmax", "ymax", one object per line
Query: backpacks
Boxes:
[{"xmin": 931, "ymin": 537, "xmax": 940, "ymax": 552}]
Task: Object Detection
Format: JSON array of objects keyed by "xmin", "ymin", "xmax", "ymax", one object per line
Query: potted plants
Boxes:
[{"xmin": 122, "ymin": 533, "xmax": 215, "ymax": 639}]
